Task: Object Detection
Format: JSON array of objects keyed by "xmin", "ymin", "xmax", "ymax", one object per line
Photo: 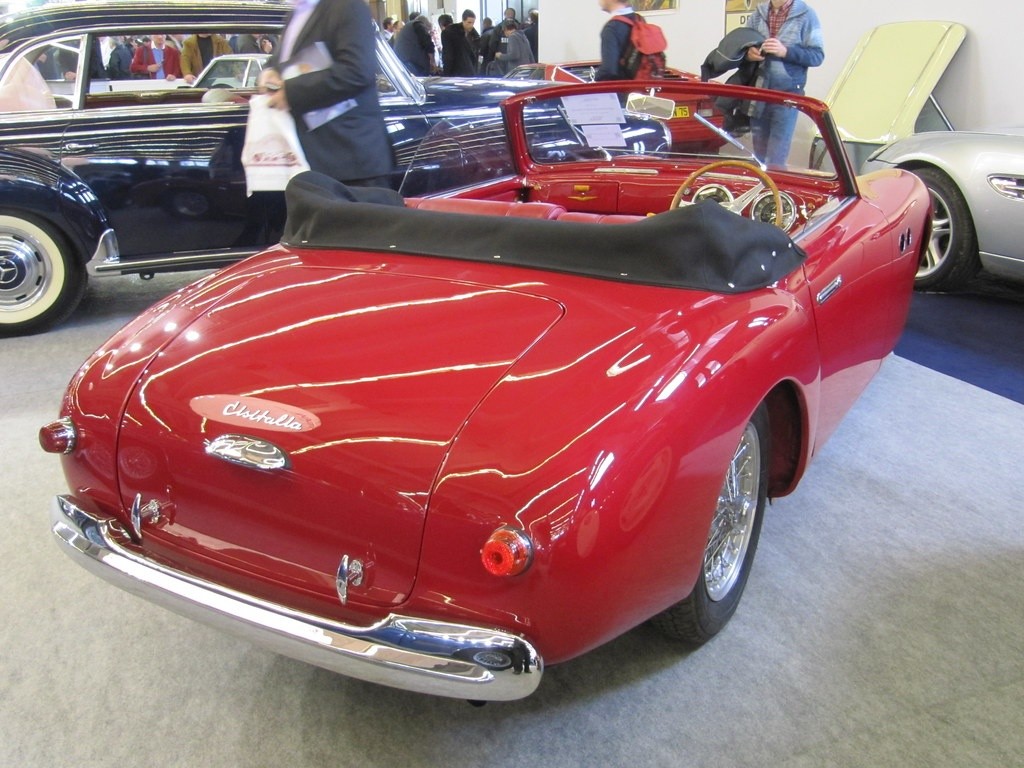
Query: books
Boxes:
[{"xmin": 279, "ymin": 41, "xmax": 357, "ymax": 131}]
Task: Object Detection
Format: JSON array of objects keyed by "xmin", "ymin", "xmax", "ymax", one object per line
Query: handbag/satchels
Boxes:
[
  {"xmin": 132, "ymin": 72, "xmax": 152, "ymax": 80},
  {"xmin": 241, "ymin": 94, "xmax": 310, "ymax": 197}
]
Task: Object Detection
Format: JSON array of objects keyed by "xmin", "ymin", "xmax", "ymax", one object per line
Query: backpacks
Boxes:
[{"xmin": 609, "ymin": 13, "xmax": 667, "ymax": 80}]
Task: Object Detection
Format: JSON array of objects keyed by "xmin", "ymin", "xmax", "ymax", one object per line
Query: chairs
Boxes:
[{"xmin": 201, "ymin": 89, "xmax": 246, "ymax": 103}]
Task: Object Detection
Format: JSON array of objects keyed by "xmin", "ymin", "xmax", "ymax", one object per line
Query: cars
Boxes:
[
  {"xmin": 494, "ymin": 61, "xmax": 730, "ymax": 158},
  {"xmin": 40, "ymin": 76, "xmax": 933, "ymax": 704},
  {"xmin": 0, "ymin": 0, "xmax": 672, "ymax": 337},
  {"xmin": 860, "ymin": 132, "xmax": 1024, "ymax": 293}
]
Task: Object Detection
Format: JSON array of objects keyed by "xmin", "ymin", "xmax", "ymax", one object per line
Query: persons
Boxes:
[
  {"xmin": 0, "ymin": 0, "xmax": 280, "ymax": 85},
  {"xmin": 479, "ymin": 8, "xmax": 539, "ymax": 77},
  {"xmin": 383, "ymin": 12, "xmax": 439, "ymax": 77},
  {"xmin": 255, "ymin": 0, "xmax": 395, "ymax": 187},
  {"xmin": 438, "ymin": 10, "xmax": 480, "ymax": 76},
  {"xmin": 745, "ymin": 0, "xmax": 825, "ymax": 164},
  {"xmin": 595, "ymin": 0, "xmax": 646, "ymax": 80}
]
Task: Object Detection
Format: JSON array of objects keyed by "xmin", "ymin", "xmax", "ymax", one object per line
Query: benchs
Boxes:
[{"xmin": 405, "ymin": 197, "xmax": 651, "ymax": 223}]
